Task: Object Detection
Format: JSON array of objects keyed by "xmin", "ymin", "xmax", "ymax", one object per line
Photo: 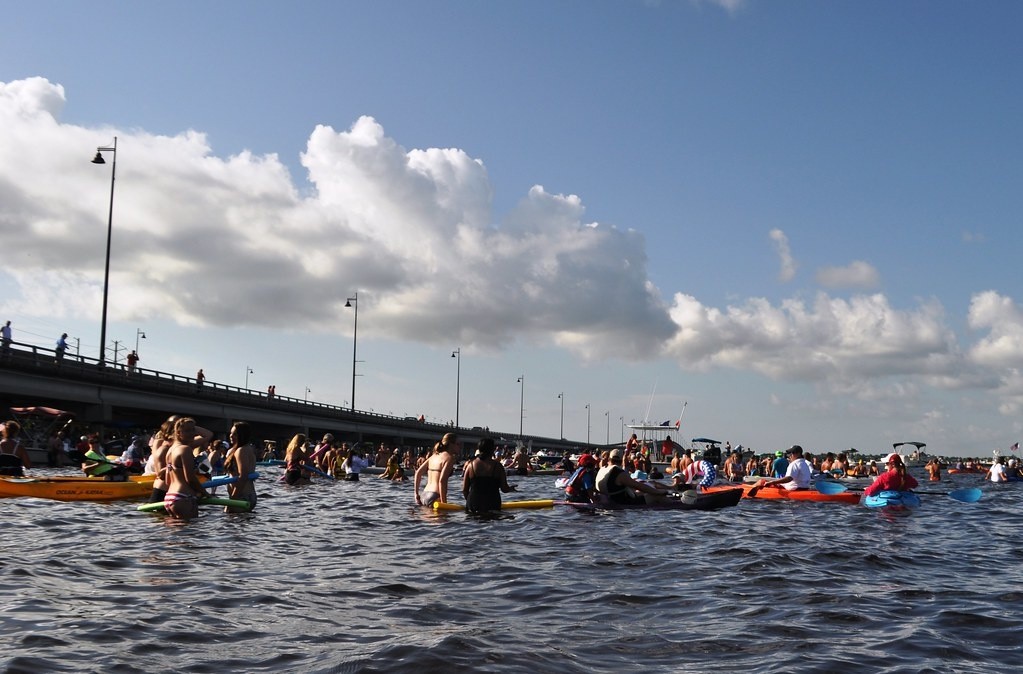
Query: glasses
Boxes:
[{"xmin": 89, "ymin": 441, "xmax": 99, "ymax": 444}]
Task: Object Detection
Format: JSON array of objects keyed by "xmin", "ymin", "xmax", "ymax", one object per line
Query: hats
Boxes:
[
  {"xmin": 786, "ymin": 445, "xmax": 803, "ymax": 454},
  {"xmin": 775, "ymin": 451, "xmax": 783, "ymax": 457},
  {"xmin": 881, "ymin": 453, "xmax": 901, "ymax": 465},
  {"xmin": 610, "ymin": 449, "xmax": 623, "ymax": 460}
]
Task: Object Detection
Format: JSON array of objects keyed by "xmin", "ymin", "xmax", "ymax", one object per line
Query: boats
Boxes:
[
  {"xmin": 893, "ymin": 441, "xmax": 926, "ymax": 468},
  {"xmin": 504, "ymin": 467, "xmax": 565, "ymax": 475},
  {"xmin": 8, "ymin": 405, "xmax": 79, "ymax": 466},
  {"xmin": 740, "ymin": 475, "xmax": 921, "ymax": 512},
  {"xmin": 642, "ymin": 486, "xmax": 745, "ymax": 510},
  {"xmin": 529, "ymin": 453, "xmax": 582, "ymax": 467},
  {"xmin": 361, "ymin": 465, "xmax": 415, "ymax": 478},
  {"xmin": 0, "ymin": 470, "xmax": 211, "ymax": 501}
]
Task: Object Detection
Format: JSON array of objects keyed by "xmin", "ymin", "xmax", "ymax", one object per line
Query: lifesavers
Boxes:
[
  {"xmin": 138, "ymin": 498, "xmax": 250, "ymax": 512},
  {"xmin": 432, "ymin": 500, "xmax": 553, "ymax": 509},
  {"xmin": 255, "ymin": 461, "xmax": 270, "ymax": 465},
  {"xmin": 268, "ymin": 459, "xmax": 334, "ymax": 481},
  {"xmin": 212, "ymin": 475, "xmax": 230, "ymax": 480},
  {"xmin": 199, "ymin": 472, "xmax": 260, "ymax": 488},
  {"xmin": 682, "ymin": 460, "xmax": 718, "ymax": 491},
  {"xmin": 279, "ymin": 444, "xmax": 329, "ymax": 480}
]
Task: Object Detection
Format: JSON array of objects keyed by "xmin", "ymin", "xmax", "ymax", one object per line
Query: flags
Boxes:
[{"xmin": 1009, "ymin": 443, "xmax": 1020, "ymax": 452}]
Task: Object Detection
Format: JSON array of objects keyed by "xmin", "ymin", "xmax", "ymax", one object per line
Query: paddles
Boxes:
[
  {"xmin": 748, "ymin": 484, "xmax": 765, "ymax": 498},
  {"xmin": 815, "ymin": 480, "xmax": 984, "ymax": 503},
  {"xmin": 666, "ymin": 489, "xmax": 698, "ymax": 505},
  {"xmin": 66, "ymin": 449, "xmax": 141, "ymax": 474}
]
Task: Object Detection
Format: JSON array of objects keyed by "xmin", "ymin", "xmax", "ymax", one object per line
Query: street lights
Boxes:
[
  {"xmin": 517, "ymin": 377, "xmax": 524, "ymax": 437},
  {"xmin": 91, "ymin": 147, "xmax": 119, "ymax": 365},
  {"xmin": 246, "ymin": 368, "xmax": 254, "ymax": 393},
  {"xmin": 585, "ymin": 405, "xmax": 590, "ymax": 444},
  {"xmin": 452, "ymin": 351, "xmax": 461, "ymax": 427},
  {"xmin": 305, "ymin": 388, "xmax": 311, "ymax": 400},
  {"xmin": 345, "ymin": 297, "xmax": 358, "ymax": 409},
  {"xmin": 605, "ymin": 412, "xmax": 662, "ymax": 445},
  {"xmin": 558, "ymin": 394, "xmax": 564, "ymax": 439},
  {"xmin": 136, "ymin": 331, "xmax": 147, "ymax": 355}
]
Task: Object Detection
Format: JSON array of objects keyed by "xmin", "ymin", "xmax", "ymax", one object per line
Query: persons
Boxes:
[
  {"xmin": 985, "ymin": 456, "xmax": 1023, "ymax": 483},
  {"xmin": 127, "ymin": 350, "xmax": 139, "ymax": 377},
  {"xmin": 45, "ymin": 420, "xmax": 283, "ymax": 478},
  {"xmin": 956, "ymin": 457, "xmax": 984, "ymax": 470},
  {"xmin": 0, "ymin": 321, "xmax": 11, "ymax": 348},
  {"xmin": 0, "ymin": 421, "xmax": 30, "ymax": 476},
  {"xmin": 723, "ymin": 440, "xmax": 879, "ymax": 483},
  {"xmin": 197, "ymin": 369, "xmax": 205, "ymax": 385},
  {"xmin": 286, "ymin": 433, "xmax": 432, "ymax": 482},
  {"xmin": 56, "ymin": 333, "xmax": 69, "ymax": 358},
  {"xmin": 463, "ymin": 438, "xmax": 555, "ymax": 476},
  {"xmin": 267, "ymin": 386, "xmax": 275, "ymax": 398},
  {"xmin": 925, "ymin": 457, "xmax": 944, "ymax": 481},
  {"xmin": 144, "ymin": 415, "xmax": 213, "ymax": 519},
  {"xmin": 450, "ymin": 420, "xmax": 454, "ymax": 428},
  {"xmin": 461, "ymin": 438, "xmax": 519, "ymax": 513},
  {"xmin": 483, "ymin": 427, "xmax": 489, "ymax": 432},
  {"xmin": 763, "ymin": 445, "xmax": 811, "ymax": 491},
  {"xmin": 564, "ymin": 434, "xmax": 722, "ymax": 479},
  {"xmin": 865, "ymin": 454, "xmax": 918, "ymax": 497},
  {"xmin": 564, "ymin": 448, "xmax": 685, "ymax": 509},
  {"xmin": 913, "ymin": 451, "xmax": 918, "ymax": 458},
  {"xmin": 223, "ymin": 421, "xmax": 258, "ymax": 514},
  {"xmin": 414, "ymin": 433, "xmax": 461, "ymax": 509},
  {"xmin": 419, "ymin": 415, "xmax": 425, "ymax": 423}
]
